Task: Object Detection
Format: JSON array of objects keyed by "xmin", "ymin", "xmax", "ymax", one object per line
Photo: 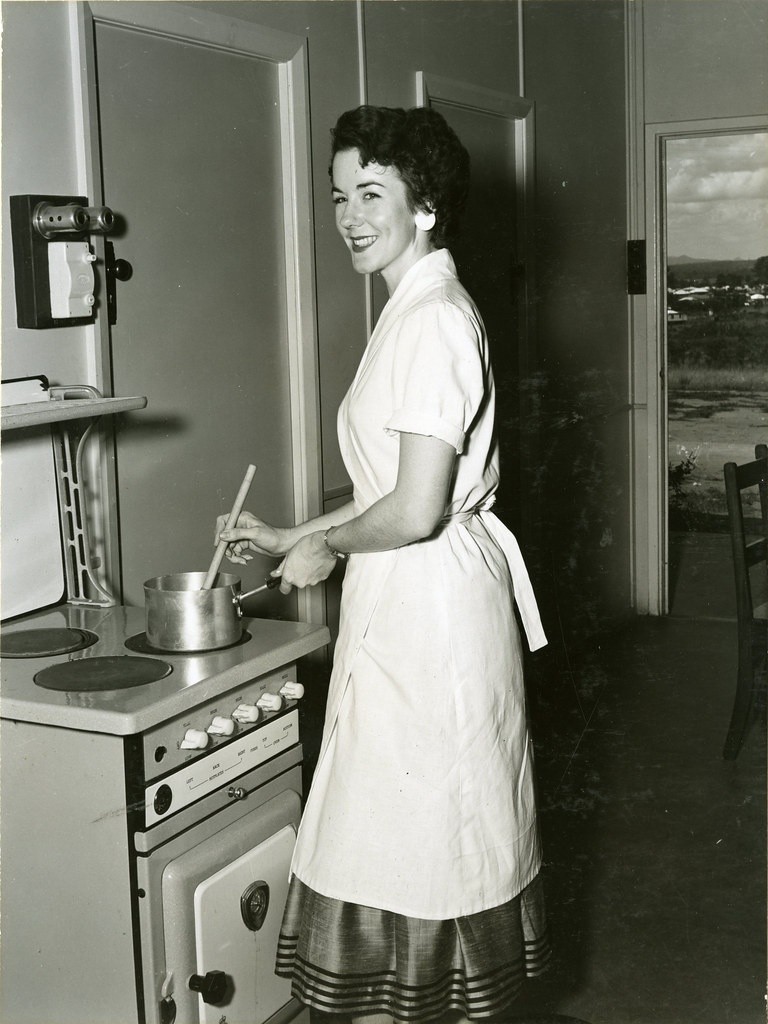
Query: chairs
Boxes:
[{"xmin": 723, "ymin": 444, "xmax": 768, "ymax": 762}]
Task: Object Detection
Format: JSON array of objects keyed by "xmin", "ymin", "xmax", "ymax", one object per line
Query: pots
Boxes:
[{"xmin": 143, "ymin": 558, "xmax": 281, "ymax": 651}]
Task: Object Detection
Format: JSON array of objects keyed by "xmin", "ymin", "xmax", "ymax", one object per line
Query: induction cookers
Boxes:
[{"xmin": 0, "ymin": 604, "xmax": 331, "ymax": 832}]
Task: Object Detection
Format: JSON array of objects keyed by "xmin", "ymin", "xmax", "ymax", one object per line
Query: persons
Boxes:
[{"xmin": 210, "ymin": 102, "xmax": 555, "ymax": 1023}]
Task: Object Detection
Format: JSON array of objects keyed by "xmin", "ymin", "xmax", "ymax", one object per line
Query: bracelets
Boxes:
[{"xmin": 323, "ymin": 524, "xmax": 353, "ymax": 560}]
